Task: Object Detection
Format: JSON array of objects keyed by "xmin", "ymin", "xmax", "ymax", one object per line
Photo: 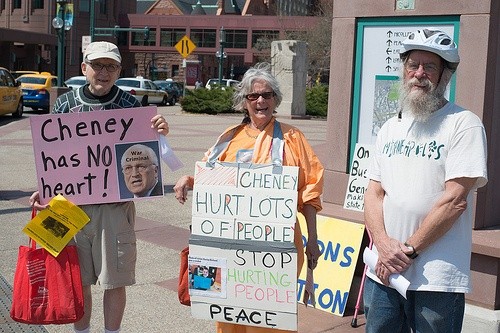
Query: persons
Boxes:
[
  {"xmin": 173, "ymin": 63, "xmax": 324, "ymax": 333},
  {"xmin": 362, "ymin": 28, "xmax": 488, "ymax": 333},
  {"xmin": 192, "ymin": 268, "xmax": 215, "ymax": 290},
  {"xmin": 194, "ymin": 79, "xmax": 203, "ymax": 89},
  {"xmin": 121, "ymin": 143, "xmax": 163, "ymax": 198},
  {"xmin": 29, "ymin": 42, "xmax": 169, "ymax": 333}
]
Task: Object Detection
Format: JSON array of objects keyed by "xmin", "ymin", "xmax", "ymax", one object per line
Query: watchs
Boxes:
[{"xmin": 402, "ymin": 242, "xmax": 418, "ymax": 259}]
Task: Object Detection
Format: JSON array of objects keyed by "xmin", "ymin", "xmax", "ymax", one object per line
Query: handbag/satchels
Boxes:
[
  {"xmin": 9, "ymin": 199, "xmax": 84, "ymax": 325},
  {"xmin": 178, "ymin": 246, "xmax": 192, "ymax": 307}
]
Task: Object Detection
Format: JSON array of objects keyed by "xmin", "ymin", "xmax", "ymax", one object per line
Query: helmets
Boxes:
[{"xmin": 400, "ymin": 29, "xmax": 459, "ymax": 63}]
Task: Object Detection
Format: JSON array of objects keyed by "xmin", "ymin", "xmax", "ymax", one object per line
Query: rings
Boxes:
[{"xmin": 174, "ymin": 195, "xmax": 176, "ymax": 197}]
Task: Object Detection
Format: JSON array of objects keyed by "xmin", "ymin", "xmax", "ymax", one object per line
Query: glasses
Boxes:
[
  {"xmin": 404, "ymin": 62, "xmax": 443, "ymax": 73},
  {"xmin": 84, "ymin": 59, "xmax": 120, "ymax": 72},
  {"xmin": 243, "ymin": 90, "xmax": 277, "ymax": 101},
  {"xmin": 122, "ymin": 163, "xmax": 148, "ymax": 174}
]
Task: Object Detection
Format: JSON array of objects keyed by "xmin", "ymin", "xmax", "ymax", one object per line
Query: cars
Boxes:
[
  {"xmin": 64, "ymin": 76, "xmax": 87, "ymax": 89},
  {"xmin": 114, "ymin": 76, "xmax": 168, "ymax": 107},
  {"xmin": 0, "ymin": 67, "xmax": 23, "ymax": 119},
  {"xmin": 205, "ymin": 79, "xmax": 240, "ymax": 91},
  {"xmin": 16, "ymin": 72, "xmax": 72, "ymax": 112},
  {"xmin": 153, "ymin": 78, "xmax": 190, "ymax": 106}
]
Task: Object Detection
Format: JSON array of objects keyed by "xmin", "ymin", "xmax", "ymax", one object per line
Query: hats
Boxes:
[{"xmin": 83, "ymin": 41, "xmax": 122, "ymax": 64}]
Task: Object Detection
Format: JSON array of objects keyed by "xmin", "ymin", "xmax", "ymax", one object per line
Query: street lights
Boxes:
[
  {"xmin": 215, "ymin": 25, "xmax": 228, "ymax": 90},
  {"xmin": 52, "ymin": 0, "xmax": 72, "ymax": 88}
]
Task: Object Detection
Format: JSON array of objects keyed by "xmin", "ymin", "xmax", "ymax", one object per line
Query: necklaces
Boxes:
[{"xmin": 245, "ymin": 124, "xmax": 259, "ymax": 138}]
